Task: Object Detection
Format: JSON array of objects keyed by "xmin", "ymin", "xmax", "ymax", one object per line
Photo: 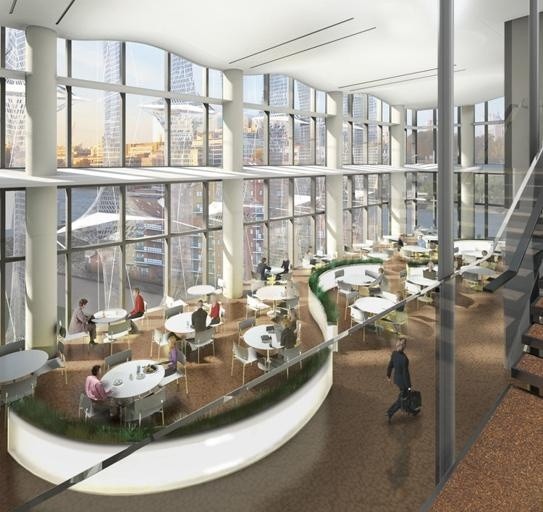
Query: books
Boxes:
[
  {"xmin": 266, "ymin": 326, "xmax": 274, "ymax": 334},
  {"xmin": 261, "ymin": 335, "xmax": 270, "ymax": 343}
]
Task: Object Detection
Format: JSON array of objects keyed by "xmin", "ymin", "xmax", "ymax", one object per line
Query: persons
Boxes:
[
  {"xmin": 159, "ymin": 332, "xmax": 177, "ymax": 377},
  {"xmin": 256, "ymin": 257, "xmax": 271, "ymax": 280},
  {"xmin": 208, "ymin": 295, "xmax": 220, "ymax": 328},
  {"xmin": 398, "ymin": 233, "xmax": 426, "ymax": 251},
  {"xmin": 126, "ymin": 288, "xmax": 144, "ymax": 333},
  {"xmin": 189, "ymin": 300, "xmax": 207, "ymax": 335},
  {"xmin": 482, "ymin": 250, "xmax": 493, "ymax": 262},
  {"xmin": 280, "ymin": 308, "xmax": 298, "ymax": 348},
  {"xmin": 372, "ymin": 268, "xmax": 384, "ymax": 286},
  {"xmin": 85, "ymin": 365, "xmax": 118, "ymax": 418},
  {"xmin": 385, "ymin": 338, "xmax": 421, "ymax": 421},
  {"xmin": 69, "ymin": 299, "xmax": 98, "ymax": 345},
  {"xmin": 276, "ymin": 253, "xmax": 289, "ymax": 280}
]
